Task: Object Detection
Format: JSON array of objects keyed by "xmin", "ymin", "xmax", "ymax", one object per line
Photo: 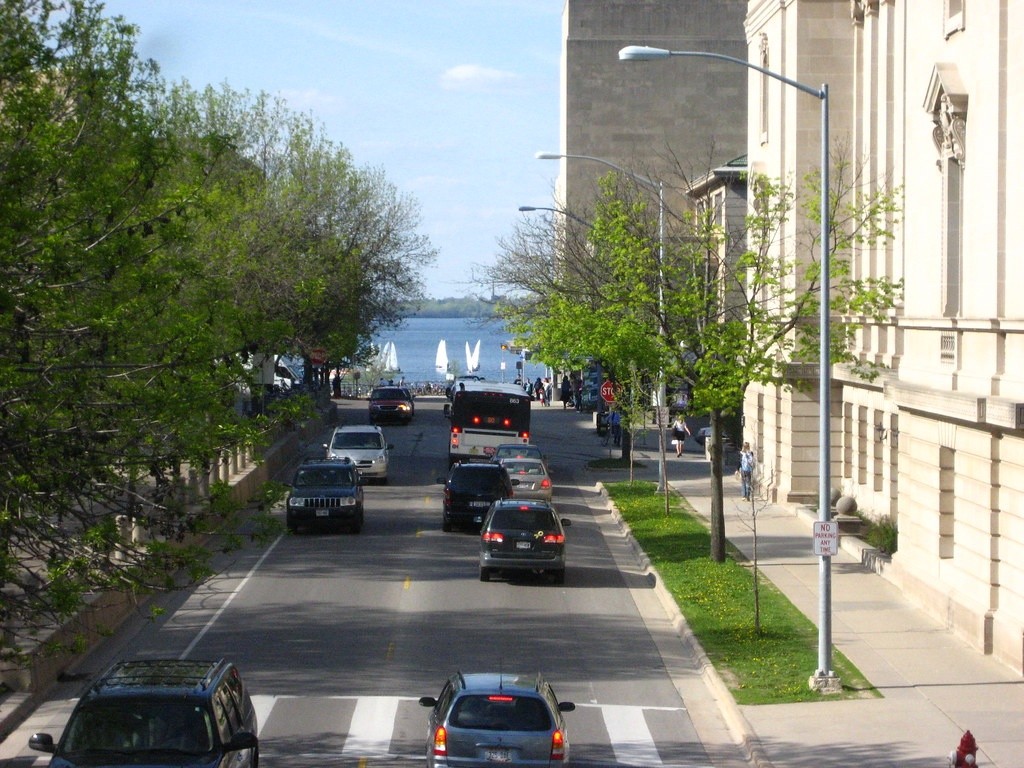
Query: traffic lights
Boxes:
[{"xmin": 501, "ymin": 343, "xmax": 506, "ymax": 350}]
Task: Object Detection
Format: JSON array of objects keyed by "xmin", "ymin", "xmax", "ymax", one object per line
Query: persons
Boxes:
[
  {"xmin": 389, "ymin": 380, "xmax": 395, "ymax": 386},
  {"xmin": 672, "ymin": 415, "xmax": 691, "ymax": 457},
  {"xmin": 735, "ymin": 442, "xmax": 753, "ymax": 501},
  {"xmin": 539, "ymin": 378, "xmax": 550, "ymax": 407},
  {"xmin": 514, "ymin": 374, "xmax": 521, "ymax": 385},
  {"xmin": 534, "ymin": 377, "xmax": 543, "ymax": 400},
  {"xmin": 559, "ymin": 373, "xmax": 582, "ymax": 410},
  {"xmin": 524, "ymin": 378, "xmax": 533, "ymax": 396},
  {"xmin": 378, "ymin": 378, "xmax": 387, "ymax": 386},
  {"xmin": 400, "ymin": 377, "xmax": 405, "ymax": 386}
]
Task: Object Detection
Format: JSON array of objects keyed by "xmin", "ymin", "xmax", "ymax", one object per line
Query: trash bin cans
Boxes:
[{"xmin": 596, "ymin": 412, "xmax": 609, "ymax": 436}]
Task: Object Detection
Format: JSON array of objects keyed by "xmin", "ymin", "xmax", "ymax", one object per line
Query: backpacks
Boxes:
[{"xmin": 740, "ymin": 451, "xmax": 753, "ymax": 472}]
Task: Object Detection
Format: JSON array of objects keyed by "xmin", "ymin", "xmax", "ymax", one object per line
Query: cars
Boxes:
[
  {"xmin": 366, "ymin": 385, "xmax": 416, "ymax": 425},
  {"xmin": 490, "ymin": 444, "xmax": 550, "ymax": 466},
  {"xmin": 501, "ymin": 457, "xmax": 554, "ymax": 502}
]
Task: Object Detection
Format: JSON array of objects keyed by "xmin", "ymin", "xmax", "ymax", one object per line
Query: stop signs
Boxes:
[
  {"xmin": 310, "ymin": 348, "xmax": 327, "ymax": 365},
  {"xmin": 601, "ymin": 381, "xmax": 622, "ymax": 402}
]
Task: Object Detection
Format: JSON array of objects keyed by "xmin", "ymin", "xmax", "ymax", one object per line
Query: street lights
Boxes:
[
  {"xmin": 537, "ymin": 152, "xmax": 667, "ymax": 492},
  {"xmin": 620, "ymin": 45, "xmax": 832, "ymax": 678}
]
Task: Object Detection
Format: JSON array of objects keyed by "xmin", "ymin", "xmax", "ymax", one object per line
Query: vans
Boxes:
[{"xmin": 232, "ymin": 351, "xmax": 299, "ymax": 395}]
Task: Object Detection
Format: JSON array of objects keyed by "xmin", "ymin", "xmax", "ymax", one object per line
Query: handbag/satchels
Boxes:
[
  {"xmin": 735, "ymin": 471, "xmax": 740, "ymax": 481},
  {"xmin": 671, "ymin": 426, "xmax": 677, "ymax": 436}
]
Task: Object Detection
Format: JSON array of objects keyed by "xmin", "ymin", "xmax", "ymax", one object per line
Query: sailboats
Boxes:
[
  {"xmin": 369, "ymin": 341, "xmax": 402, "ymax": 374},
  {"xmin": 434, "ymin": 340, "xmax": 480, "ymax": 375}
]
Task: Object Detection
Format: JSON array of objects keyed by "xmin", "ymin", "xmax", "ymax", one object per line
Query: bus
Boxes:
[{"xmin": 444, "ymin": 376, "xmax": 536, "ymax": 465}]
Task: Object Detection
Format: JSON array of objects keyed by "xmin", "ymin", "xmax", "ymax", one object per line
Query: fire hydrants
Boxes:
[{"xmin": 947, "ymin": 729, "xmax": 978, "ymax": 768}]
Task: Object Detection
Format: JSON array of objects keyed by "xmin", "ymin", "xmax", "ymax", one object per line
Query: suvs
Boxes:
[
  {"xmin": 322, "ymin": 424, "xmax": 395, "ymax": 485},
  {"xmin": 474, "ymin": 496, "xmax": 572, "ymax": 585},
  {"xmin": 28, "ymin": 658, "xmax": 258, "ymax": 768},
  {"xmin": 419, "ymin": 668, "xmax": 576, "ymax": 768},
  {"xmin": 437, "ymin": 461, "xmax": 520, "ymax": 532},
  {"xmin": 282, "ymin": 456, "xmax": 366, "ymax": 535}
]
